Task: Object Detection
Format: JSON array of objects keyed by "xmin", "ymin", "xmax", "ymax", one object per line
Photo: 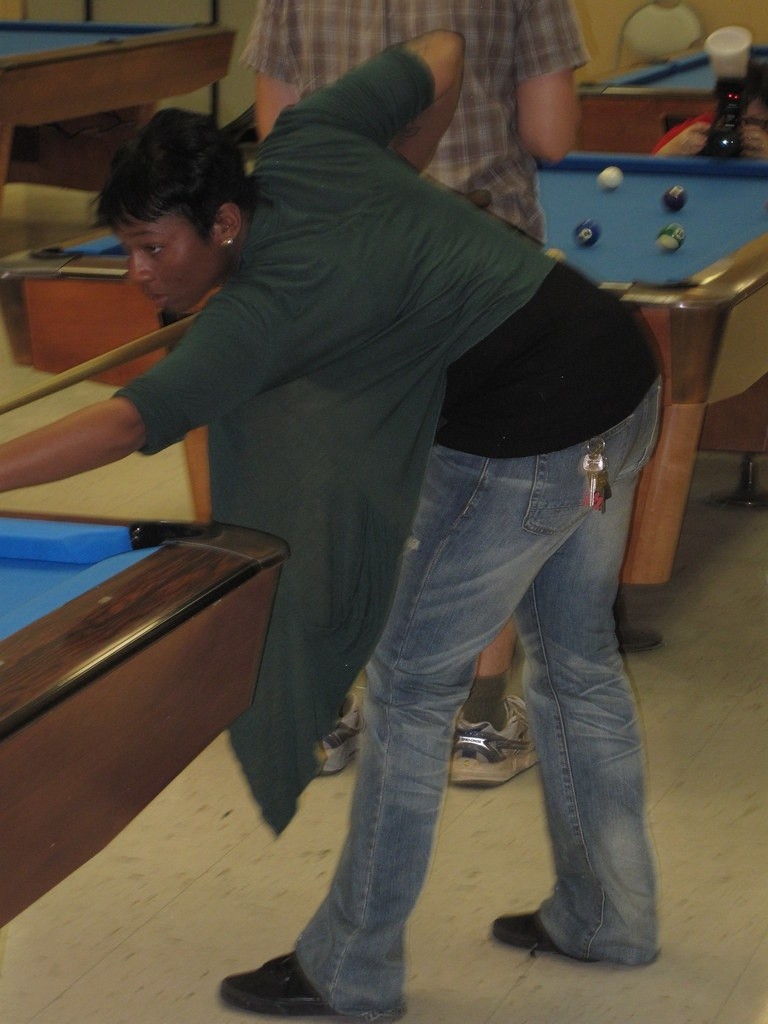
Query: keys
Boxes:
[{"xmin": 578, "ymin": 453, "xmax": 609, "ymax": 515}]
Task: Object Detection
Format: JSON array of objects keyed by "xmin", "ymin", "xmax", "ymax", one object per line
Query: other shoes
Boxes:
[{"xmin": 615, "ymin": 625, "xmax": 663, "ymax": 649}]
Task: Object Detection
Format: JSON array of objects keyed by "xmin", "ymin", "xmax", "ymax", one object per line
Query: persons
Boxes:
[
  {"xmin": 0, "ymin": 30, "xmax": 663, "ymax": 1019},
  {"xmin": 241, "ymin": 0, "xmax": 591, "ymax": 791}
]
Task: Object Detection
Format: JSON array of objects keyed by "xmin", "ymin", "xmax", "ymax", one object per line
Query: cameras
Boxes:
[{"xmin": 699, "ymin": 78, "xmax": 748, "ymax": 158}]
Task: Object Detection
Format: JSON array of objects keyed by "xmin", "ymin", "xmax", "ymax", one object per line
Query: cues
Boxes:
[{"xmin": 0, "ymin": 310, "xmax": 202, "ymax": 419}]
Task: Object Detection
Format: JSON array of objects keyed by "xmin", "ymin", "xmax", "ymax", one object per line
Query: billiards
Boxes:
[
  {"xmin": 597, "ymin": 166, "xmax": 625, "ymax": 188},
  {"xmin": 656, "ymin": 223, "xmax": 685, "ymax": 252},
  {"xmin": 574, "ymin": 219, "xmax": 602, "ymax": 248},
  {"xmin": 663, "ymin": 184, "xmax": 688, "ymax": 212}
]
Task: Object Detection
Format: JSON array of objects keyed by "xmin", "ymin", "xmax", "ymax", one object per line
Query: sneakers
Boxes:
[
  {"xmin": 316, "ymin": 693, "xmax": 363, "ymax": 775},
  {"xmin": 493, "ymin": 909, "xmax": 595, "ymax": 964},
  {"xmin": 452, "ymin": 695, "xmax": 537, "ymax": 785},
  {"xmin": 220, "ymin": 951, "xmax": 340, "ymax": 1016}
]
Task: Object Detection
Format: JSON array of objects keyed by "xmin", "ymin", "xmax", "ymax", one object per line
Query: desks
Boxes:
[
  {"xmin": 0, "ymin": 508, "xmax": 290, "ymax": 928},
  {"xmin": 0, "ymin": 20, "xmax": 241, "ymax": 205},
  {"xmin": 574, "ymin": 45, "xmax": 768, "ymax": 153},
  {"xmin": 0, "ymin": 151, "xmax": 768, "ymax": 652}
]
